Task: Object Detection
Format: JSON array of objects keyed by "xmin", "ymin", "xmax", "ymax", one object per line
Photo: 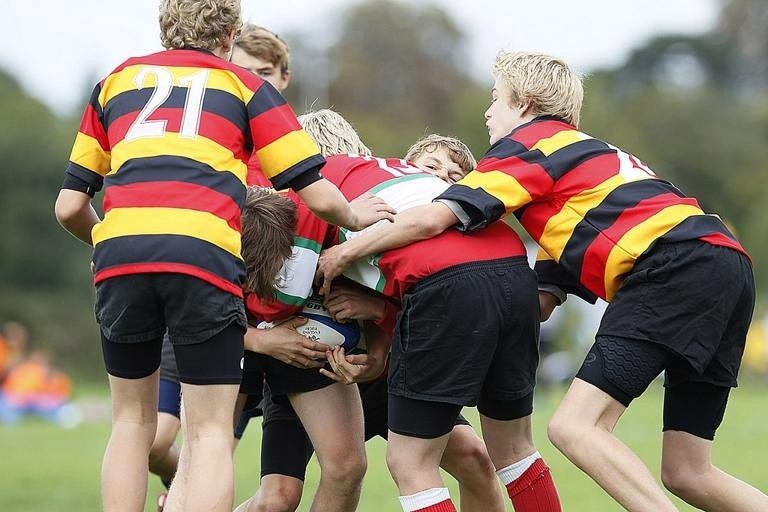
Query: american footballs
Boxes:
[{"xmin": 254, "ymin": 296, "xmax": 361, "ymax": 364}]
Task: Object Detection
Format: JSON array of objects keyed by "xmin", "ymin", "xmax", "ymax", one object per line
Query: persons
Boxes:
[
  {"xmin": 310, "ymin": 50, "xmax": 768, "ymax": 512},
  {"xmin": 0, "ymin": 313, "xmax": 94, "ymax": 432},
  {"xmin": 148, "ymin": 22, "xmax": 561, "ymax": 511},
  {"xmin": 55, "ymin": 0, "xmax": 397, "ymax": 512}
]
